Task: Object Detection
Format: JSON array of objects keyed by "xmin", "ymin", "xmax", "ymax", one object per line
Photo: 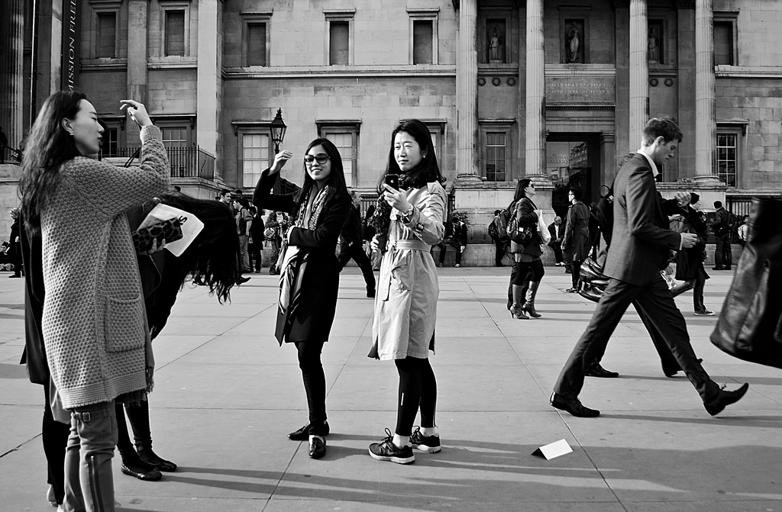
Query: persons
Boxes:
[
  {"xmin": 215, "ymin": 187, "xmax": 468, "ymax": 270},
  {"xmin": 368, "ymin": 117, "xmax": 448, "ymax": 464},
  {"xmin": 493, "ymin": 179, "xmax": 545, "ymax": 319},
  {"xmin": 254, "ymin": 137, "xmax": 360, "ymax": 459},
  {"xmin": 9, "ymin": 91, "xmax": 171, "ymax": 512},
  {"xmin": 547, "ymin": 116, "xmax": 749, "ymax": 417}
]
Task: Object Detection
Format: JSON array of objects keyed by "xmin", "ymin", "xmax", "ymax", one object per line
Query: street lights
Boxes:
[{"xmin": 267, "ymin": 108, "xmax": 287, "ymax": 274}]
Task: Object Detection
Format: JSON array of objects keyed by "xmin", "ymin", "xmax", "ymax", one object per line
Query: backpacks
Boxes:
[{"xmin": 488, "ymin": 218, "xmax": 499, "ymax": 244}]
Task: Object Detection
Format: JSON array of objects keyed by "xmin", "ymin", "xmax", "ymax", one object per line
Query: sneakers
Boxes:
[
  {"xmin": 460, "ymin": 246, "xmax": 465, "ymax": 253},
  {"xmin": 409, "ymin": 426, "xmax": 441, "ymax": 453},
  {"xmin": 455, "ymin": 264, "xmax": 460, "ymax": 267},
  {"xmin": 368, "ymin": 428, "xmax": 415, "ymax": 464},
  {"xmin": 694, "ymin": 309, "xmax": 715, "ymax": 316}
]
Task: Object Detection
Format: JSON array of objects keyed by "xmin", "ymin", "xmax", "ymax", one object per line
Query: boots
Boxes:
[
  {"xmin": 510, "ymin": 284, "xmax": 529, "ymax": 319},
  {"xmin": 523, "ymin": 281, "xmax": 541, "ymax": 317}
]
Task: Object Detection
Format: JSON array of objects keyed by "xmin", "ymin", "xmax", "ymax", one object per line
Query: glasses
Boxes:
[
  {"xmin": 305, "ymin": 153, "xmax": 330, "ymax": 165},
  {"xmin": 529, "ymin": 184, "xmax": 534, "ymax": 187}
]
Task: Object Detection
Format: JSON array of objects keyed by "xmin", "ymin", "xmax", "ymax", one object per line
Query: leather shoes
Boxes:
[
  {"xmin": 236, "ymin": 277, "xmax": 251, "ymax": 285},
  {"xmin": 121, "ymin": 462, "xmax": 162, "ymax": 482},
  {"xmin": 137, "ymin": 451, "xmax": 177, "ymax": 472},
  {"xmin": 723, "ymin": 265, "xmax": 731, "ymax": 270},
  {"xmin": 309, "ymin": 436, "xmax": 326, "ymax": 459},
  {"xmin": 287, "ymin": 422, "xmax": 329, "ymax": 440},
  {"xmin": 712, "ymin": 266, "xmax": 723, "ymax": 270},
  {"xmin": 566, "ymin": 288, "xmax": 578, "ymax": 294},
  {"xmin": 548, "ymin": 391, "xmax": 600, "ymax": 417},
  {"xmin": 661, "ymin": 358, "xmax": 703, "ymax": 377},
  {"xmin": 585, "ymin": 364, "xmax": 619, "ymax": 378},
  {"xmin": 703, "ymin": 383, "xmax": 748, "ymax": 416}
]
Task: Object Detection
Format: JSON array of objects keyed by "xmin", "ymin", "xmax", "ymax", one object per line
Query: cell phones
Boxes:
[{"xmin": 385, "ymin": 174, "xmax": 398, "ymax": 194}]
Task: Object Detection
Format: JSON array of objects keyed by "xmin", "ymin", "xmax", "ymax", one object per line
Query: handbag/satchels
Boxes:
[
  {"xmin": 507, "ymin": 203, "xmax": 533, "ymax": 244},
  {"xmin": 576, "ymin": 256, "xmax": 613, "ymax": 303},
  {"xmin": 534, "ymin": 210, "xmax": 551, "ymax": 246},
  {"xmin": 709, "ymin": 195, "xmax": 779, "ymax": 369},
  {"xmin": 263, "ymin": 227, "xmax": 276, "ymax": 240},
  {"xmin": 132, "ymin": 217, "xmax": 187, "ymax": 252}
]
